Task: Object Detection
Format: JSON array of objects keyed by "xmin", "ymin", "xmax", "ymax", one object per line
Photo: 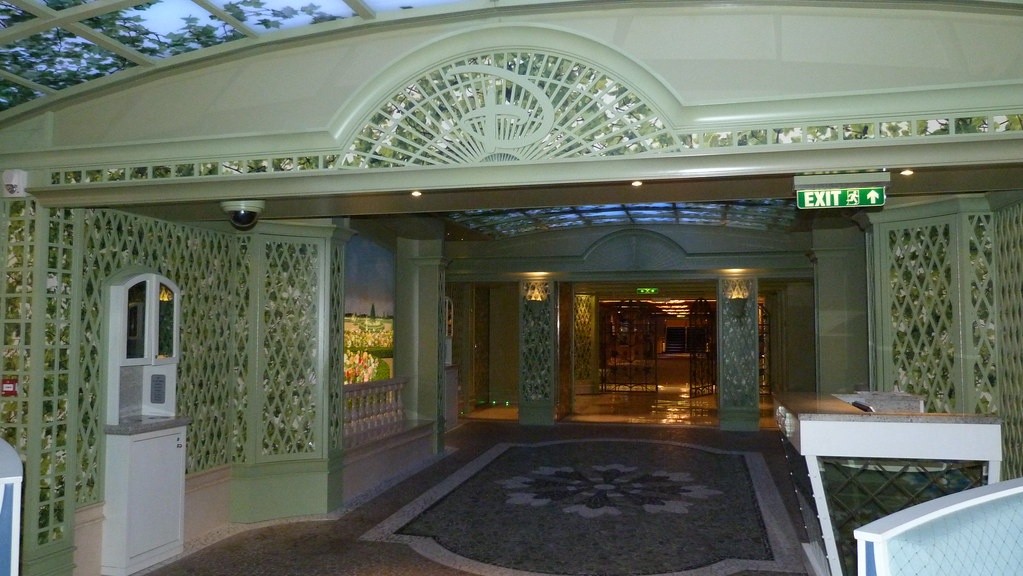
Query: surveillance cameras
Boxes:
[{"xmin": 220, "ymin": 200, "xmax": 265, "ymax": 231}]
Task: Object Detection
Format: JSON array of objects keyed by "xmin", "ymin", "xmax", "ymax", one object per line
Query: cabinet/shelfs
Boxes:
[{"xmin": 100, "ymin": 425, "xmax": 187, "ymax": 576}]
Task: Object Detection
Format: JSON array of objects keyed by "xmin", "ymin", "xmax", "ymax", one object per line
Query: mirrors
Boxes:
[{"xmin": 101, "ymin": 263, "xmax": 181, "ymax": 367}]
